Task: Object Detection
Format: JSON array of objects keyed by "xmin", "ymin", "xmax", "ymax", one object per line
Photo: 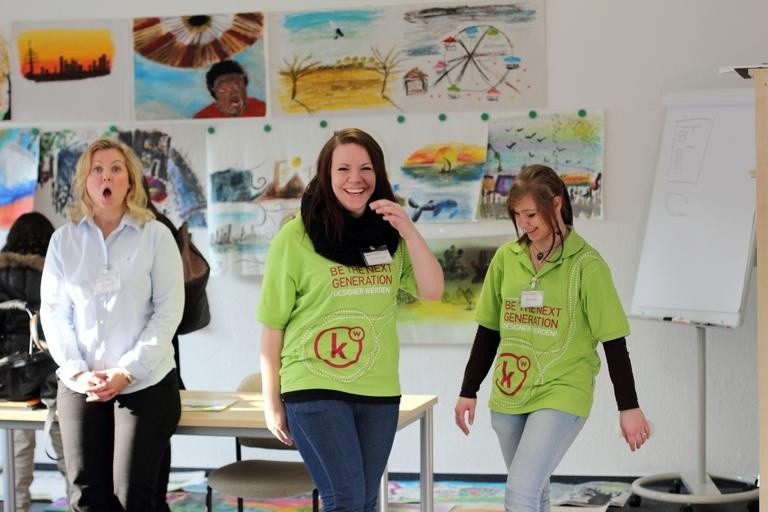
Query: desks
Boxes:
[{"xmin": 0, "ymin": 389, "xmax": 438, "ymax": 512}]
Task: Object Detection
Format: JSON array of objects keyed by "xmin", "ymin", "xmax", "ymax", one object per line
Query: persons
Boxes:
[
  {"xmin": 41, "ymin": 138, "xmax": 188, "ymax": 512},
  {"xmin": 140, "ymin": 177, "xmax": 212, "ymax": 393},
  {"xmin": 192, "ymin": 59, "xmax": 266, "ymax": 117},
  {"xmin": 450, "ymin": 165, "xmax": 653, "ymax": 512},
  {"xmin": 257, "ymin": 128, "xmax": 445, "ymax": 511},
  {"xmin": 0, "ymin": 211, "xmax": 71, "ymax": 512}
]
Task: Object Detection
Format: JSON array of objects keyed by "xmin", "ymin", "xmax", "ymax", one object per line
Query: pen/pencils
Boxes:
[
  {"xmin": 663, "ymin": 317, "xmax": 690, "ymax": 324},
  {"xmin": 192, "ymin": 404, "xmax": 214, "ymax": 408}
]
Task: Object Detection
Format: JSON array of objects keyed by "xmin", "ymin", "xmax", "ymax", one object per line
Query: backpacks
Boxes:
[{"xmin": 174, "ymin": 221, "xmax": 210, "ymax": 335}]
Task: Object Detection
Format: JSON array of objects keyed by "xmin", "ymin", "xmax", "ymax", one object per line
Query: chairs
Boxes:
[{"xmin": 205, "ymin": 373, "xmax": 320, "ymax": 512}]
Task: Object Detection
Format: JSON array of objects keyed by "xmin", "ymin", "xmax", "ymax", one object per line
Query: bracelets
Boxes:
[{"xmin": 122, "ymin": 373, "xmax": 135, "ymax": 383}]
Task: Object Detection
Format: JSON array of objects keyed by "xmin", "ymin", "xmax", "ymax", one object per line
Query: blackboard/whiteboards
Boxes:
[{"xmin": 628, "ymin": 87, "xmax": 755, "ymax": 329}]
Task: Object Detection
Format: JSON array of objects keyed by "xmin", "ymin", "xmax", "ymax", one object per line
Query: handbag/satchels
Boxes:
[{"xmin": 0, "ymin": 300, "xmax": 60, "ymax": 402}]
